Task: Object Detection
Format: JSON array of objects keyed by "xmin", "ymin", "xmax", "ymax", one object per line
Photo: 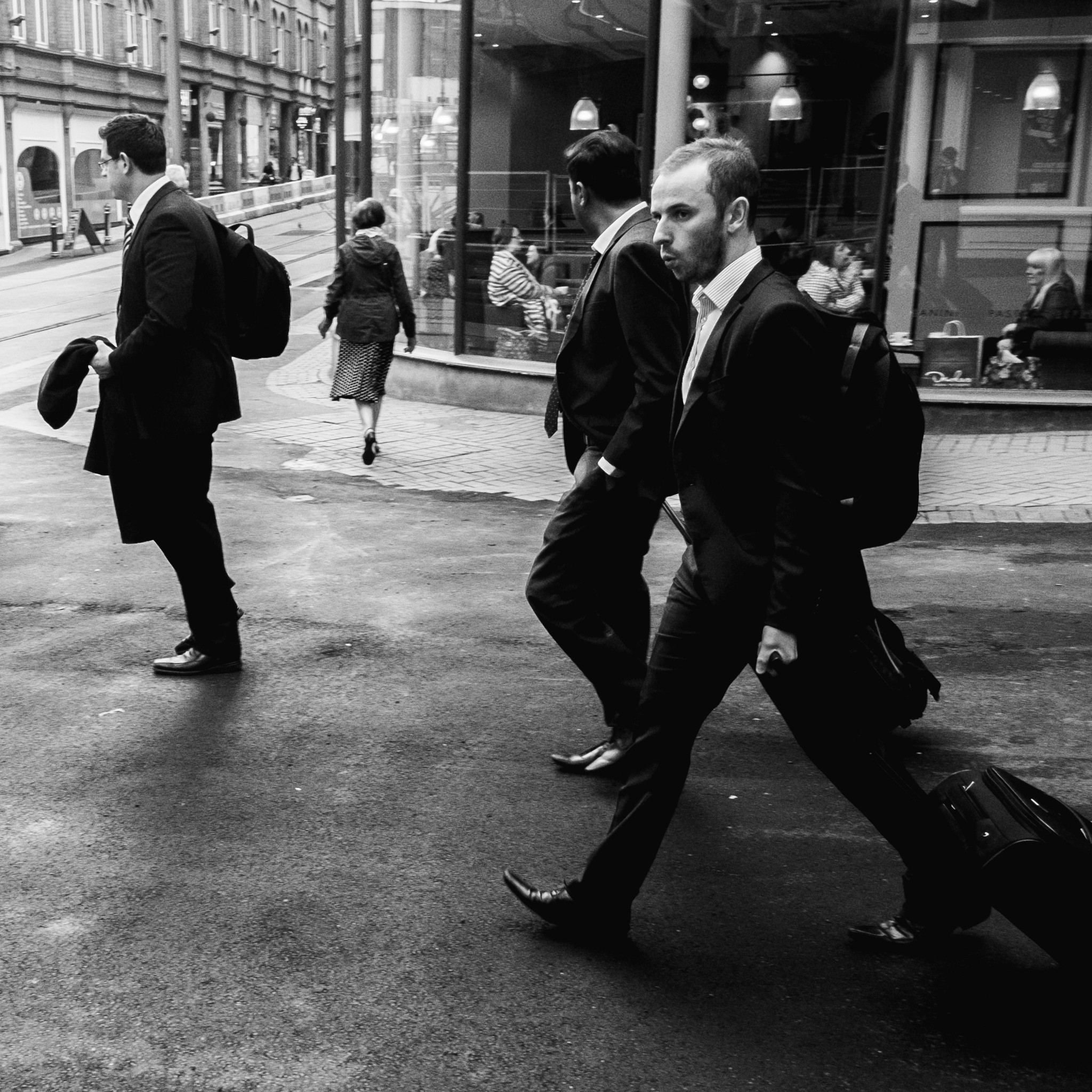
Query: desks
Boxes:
[{"xmin": 891, "ymin": 340, "xmax": 924, "ymax": 383}]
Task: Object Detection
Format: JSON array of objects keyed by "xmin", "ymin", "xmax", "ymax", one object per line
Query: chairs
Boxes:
[
  {"xmin": 1031, "ymin": 327, "xmax": 1092, "ymax": 391},
  {"xmin": 441, "ymin": 240, "xmax": 526, "ymax": 352}
]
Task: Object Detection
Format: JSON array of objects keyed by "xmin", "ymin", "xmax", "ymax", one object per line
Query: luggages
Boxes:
[
  {"xmin": 661, "ymin": 501, "xmax": 942, "ymax": 728},
  {"xmin": 753, "ymin": 641, "xmax": 1092, "ymax": 967}
]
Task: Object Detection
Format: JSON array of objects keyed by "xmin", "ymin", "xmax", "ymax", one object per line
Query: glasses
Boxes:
[{"xmin": 511, "ymin": 233, "xmax": 521, "ymax": 239}]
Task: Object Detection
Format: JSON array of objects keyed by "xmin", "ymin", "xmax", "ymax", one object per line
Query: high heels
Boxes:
[{"xmin": 362, "ymin": 428, "xmax": 380, "ymax": 465}]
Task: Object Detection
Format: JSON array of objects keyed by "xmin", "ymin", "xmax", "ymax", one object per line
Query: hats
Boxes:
[{"xmin": 37, "ymin": 339, "xmax": 97, "ymax": 429}]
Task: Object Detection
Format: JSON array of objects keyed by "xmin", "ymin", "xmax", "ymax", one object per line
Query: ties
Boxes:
[{"xmin": 121, "ymin": 215, "xmax": 134, "ymax": 279}]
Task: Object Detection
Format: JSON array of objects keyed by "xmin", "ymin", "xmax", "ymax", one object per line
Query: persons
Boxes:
[
  {"xmin": 504, "ymin": 139, "xmax": 991, "ymax": 954},
  {"xmin": 923, "ymin": 146, "xmax": 969, "ymax": 291},
  {"xmin": 759, "ymin": 210, "xmax": 810, "ymax": 276},
  {"xmin": 527, "ymin": 129, "xmax": 688, "ymax": 776},
  {"xmin": 317, "ymin": 197, "xmax": 417, "ymax": 466},
  {"xmin": 982, "ymin": 247, "xmax": 1084, "ymax": 375},
  {"xmin": 797, "ymin": 233, "xmax": 865, "ymax": 313},
  {"xmin": 489, "ymin": 223, "xmax": 569, "ymax": 331},
  {"xmin": 83, "ymin": 113, "xmax": 244, "ymax": 676},
  {"xmin": 286, "ymin": 157, "xmax": 303, "ymax": 209},
  {"xmin": 258, "ymin": 162, "xmax": 276, "ymax": 187}
]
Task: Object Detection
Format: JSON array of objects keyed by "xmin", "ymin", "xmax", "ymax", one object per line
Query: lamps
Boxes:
[
  {"xmin": 569, "ymin": 89, "xmax": 601, "ymax": 131},
  {"xmin": 767, "ymin": 76, "xmax": 803, "ymax": 122},
  {"xmin": 272, "ymin": 48, "xmax": 281, "ymax": 55},
  {"xmin": 209, "ymin": 28, "xmax": 220, "ymax": 36},
  {"xmin": 124, "ymin": 44, "xmax": 139, "ymax": 54},
  {"xmin": 1020, "ymin": 57, "xmax": 1061, "ymax": 113},
  {"xmin": 318, "ymin": 63, "xmax": 329, "ymax": 70},
  {"xmin": 8, "ymin": 14, "xmax": 25, "ymax": 26}
]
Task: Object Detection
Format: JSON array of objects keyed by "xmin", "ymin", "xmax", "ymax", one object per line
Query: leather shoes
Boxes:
[
  {"xmin": 845, "ymin": 919, "xmax": 915, "ymax": 948},
  {"xmin": 153, "ymin": 607, "xmax": 244, "ymax": 673},
  {"xmin": 503, "ymin": 867, "xmax": 638, "ymax": 941},
  {"xmin": 550, "ymin": 736, "xmax": 628, "ymax": 782}
]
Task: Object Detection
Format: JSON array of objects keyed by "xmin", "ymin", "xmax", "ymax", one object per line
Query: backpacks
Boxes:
[
  {"xmin": 812, "ymin": 293, "xmax": 926, "ymax": 550},
  {"xmin": 203, "ymin": 206, "xmax": 292, "ymax": 361}
]
